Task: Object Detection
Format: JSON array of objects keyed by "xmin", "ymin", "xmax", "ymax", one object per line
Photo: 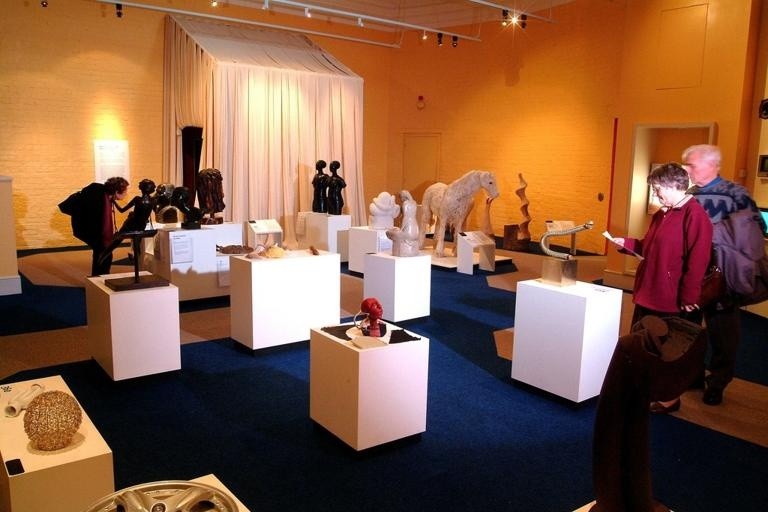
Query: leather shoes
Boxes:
[{"xmin": 702, "ymin": 383, "xmax": 724, "ymax": 406}]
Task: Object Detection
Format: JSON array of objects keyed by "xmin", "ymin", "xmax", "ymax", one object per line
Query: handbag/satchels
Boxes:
[{"xmin": 58, "ymin": 190, "xmax": 83, "ymax": 216}]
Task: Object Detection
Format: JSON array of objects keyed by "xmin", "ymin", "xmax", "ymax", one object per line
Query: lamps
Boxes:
[
  {"xmin": 40, "ymin": 1, "xmax": 49, "ymax": 8},
  {"xmin": 452, "ymin": 36, "xmax": 458, "ymax": 47},
  {"xmin": 114, "ymin": 4, "xmax": 123, "ymax": 16},
  {"xmin": 438, "ymin": 33, "xmax": 443, "ymax": 47},
  {"xmin": 502, "ymin": 9, "xmax": 508, "ymax": 19},
  {"xmin": 521, "ymin": 14, "xmax": 527, "ymax": 28}
]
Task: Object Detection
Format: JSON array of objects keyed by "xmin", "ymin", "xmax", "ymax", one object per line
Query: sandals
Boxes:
[{"xmin": 651, "ymin": 399, "xmax": 681, "ymax": 413}]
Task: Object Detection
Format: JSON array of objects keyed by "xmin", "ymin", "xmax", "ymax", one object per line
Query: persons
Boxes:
[
  {"xmin": 386, "ymin": 190, "xmax": 420, "ymax": 256},
  {"xmin": 360, "ymin": 298, "xmax": 383, "ymax": 337},
  {"xmin": 312, "ymin": 160, "xmax": 346, "ymax": 215},
  {"xmin": 71, "ymin": 177, "xmax": 129, "ymax": 276},
  {"xmin": 589, "ymin": 308, "xmax": 705, "ymax": 512},
  {"xmin": 680, "ymin": 144, "xmax": 765, "ymax": 405},
  {"xmin": 609, "ymin": 163, "xmax": 714, "ymax": 413},
  {"xmin": 167, "ymin": 187, "xmax": 204, "ymax": 229},
  {"xmin": 112, "ymin": 179, "xmax": 168, "ymax": 284}
]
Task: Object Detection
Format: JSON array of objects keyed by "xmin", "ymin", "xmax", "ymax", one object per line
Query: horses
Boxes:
[{"xmin": 418, "ymin": 169, "xmax": 500, "ymax": 257}]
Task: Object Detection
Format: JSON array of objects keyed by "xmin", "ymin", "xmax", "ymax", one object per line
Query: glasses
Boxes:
[{"xmin": 652, "ymin": 185, "xmax": 662, "ymax": 193}]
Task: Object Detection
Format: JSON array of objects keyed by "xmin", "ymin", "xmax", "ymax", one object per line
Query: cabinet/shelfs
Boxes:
[
  {"xmin": 229, "ymin": 247, "xmax": 341, "ymax": 350},
  {"xmin": 511, "ymin": 276, "xmax": 623, "ymax": 404},
  {"xmin": 365, "ymin": 249, "xmax": 430, "ymax": 322},
  {"xmin": 141, "ymin": 224, "xmax": 243, "ymax": 302},
  {"xmin": 311, "ymin": 318, "xmax": 429, "ymax": 450},
  {"xmin": 85, "ymin": 474, "xmax": 252, "ymax": 512},
  {"xmin": 349, "ymin": 226, "xmax": 398, "ymax": 273},
  {"xmin": 298, "ymin": 211, "xmax": 351, "ymax": 261},
  {"xmin": 84, "ymin": 271, "xmax": 181, "ymax": 382},
  {"xmin": 0, "ymin": 375, "xmax": 114, "ymax": 512}
]
